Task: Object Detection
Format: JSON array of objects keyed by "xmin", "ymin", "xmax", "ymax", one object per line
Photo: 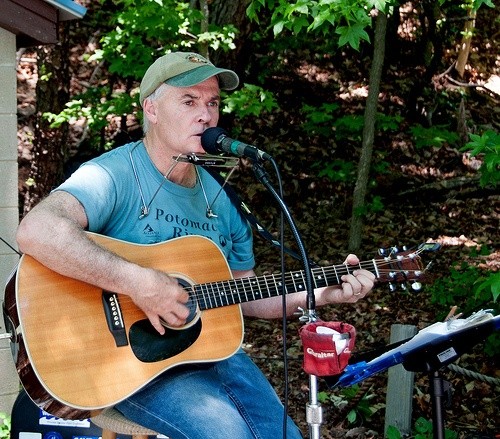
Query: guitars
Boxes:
[{"xmin": 3, "ymin": 228, "xmax": 444, "ymax": 421}]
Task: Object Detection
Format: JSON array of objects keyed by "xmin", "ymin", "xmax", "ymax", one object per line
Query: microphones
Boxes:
[{"xmin": 201, "ymin": 127, "xmax": 272, "ymax": 160}]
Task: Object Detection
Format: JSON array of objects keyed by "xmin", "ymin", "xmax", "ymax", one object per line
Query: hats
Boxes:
[{"xmin": 139, "ymin": 52, "xmax": 239, "ymax": 108}]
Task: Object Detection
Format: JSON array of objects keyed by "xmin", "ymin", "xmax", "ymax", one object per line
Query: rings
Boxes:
[{"xmin": 353, "ymin": 292, "xmax": 360, "ymax": 295}]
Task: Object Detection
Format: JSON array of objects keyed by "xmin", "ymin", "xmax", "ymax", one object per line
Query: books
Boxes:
[{"xmin": 333, "ymin": 307, "xmax": 500, "ymax": 391}]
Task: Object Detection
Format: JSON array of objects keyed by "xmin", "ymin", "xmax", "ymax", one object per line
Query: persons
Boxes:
[{"xmin": 16, "ymin": 51, "xmax": 375, "ymax": 439}]
